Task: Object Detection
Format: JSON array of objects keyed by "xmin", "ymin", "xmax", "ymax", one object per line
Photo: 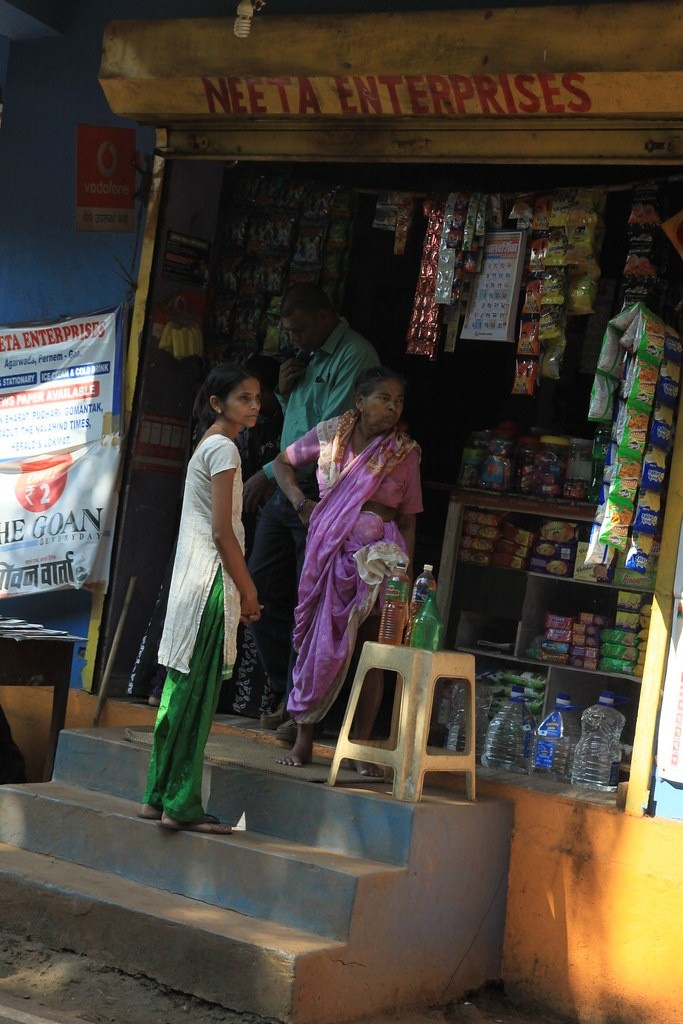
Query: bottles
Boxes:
[
  {"xmin": 446, "ymin": 670, "xmax": 494, "ymax": 763},
  {"xmin": 480, "ymin": 686, "xmax": 538, "ymax": 775},
  {"xmin": 588, "ymin": 423, "xmax": 611, "ymax": 504},
  {"xmin": 410, "ymin": 588, "xmax": 445, "ymax": 651},
  {"xmin": 405, "ymin": 565, "xmax": 437, "ymax": 647},
  {"xmin": 571, "ymin": 690, "xmax": 626, "ymax": 792},
  {"xmin": 533, "ymin": 692, "xmax": 583, "ymax": 781},
  {"xmin": 378, "ymin": 563, "xmax": 409, "ymax": 646}
]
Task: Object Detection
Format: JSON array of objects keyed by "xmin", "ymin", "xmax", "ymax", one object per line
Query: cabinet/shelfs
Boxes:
[{"xmin": 421, "ymin": 481, "xmax": 663, "ymax": 773}]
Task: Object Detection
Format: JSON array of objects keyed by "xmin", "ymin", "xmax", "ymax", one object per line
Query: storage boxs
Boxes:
[{"xmin": 573, "ymin": 536, "xmax": 660, "ymax": 589}]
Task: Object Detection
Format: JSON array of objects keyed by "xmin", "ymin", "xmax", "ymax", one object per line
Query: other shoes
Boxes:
[
  {"xmin": 261, "ymin": 702, "xmax": 289, "ymax": 729},
  {"xmin": 148, "ymin": 687, "xmax": 162, "ymax": 705},
  {"xmin": 276, "ymin": 718, "xmax": 324, "ymax": 741}
]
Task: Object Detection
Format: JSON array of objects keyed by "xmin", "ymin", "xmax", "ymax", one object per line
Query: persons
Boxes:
[
  {"xmin": 243, "ymin": 280, "xmax": 382, "ymax": 740},
  {"xmin": 271, "ymin": 367, "xmax": 425, "ymax": 778},
  {"xmin": 137, "ymin": 362, "xmax": 265, "ymax": 834}
]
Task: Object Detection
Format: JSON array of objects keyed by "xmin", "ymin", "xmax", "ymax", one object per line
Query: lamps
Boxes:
[{"xmin": 233, "ymin": 0, "xmax": 265, "ymax": 38}]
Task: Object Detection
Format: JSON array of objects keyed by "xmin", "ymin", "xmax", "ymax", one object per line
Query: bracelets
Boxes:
[{"xmin": 295, "ymin": 496, "xmax": 309, "ymax": 514}]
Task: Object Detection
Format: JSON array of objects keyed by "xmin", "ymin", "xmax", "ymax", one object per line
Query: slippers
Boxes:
[
  {"xmin": 159, "ymin": 815, "xmax": 232, "ymax": 835},
  {"xmin": 137, "ymin": 812, "xmax": 162, "ymax": 820}
]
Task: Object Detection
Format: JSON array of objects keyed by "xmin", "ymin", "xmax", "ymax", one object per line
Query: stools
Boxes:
[{"xmin": 326, "ymin": 641, "xmax": 478, "ymax": 804}]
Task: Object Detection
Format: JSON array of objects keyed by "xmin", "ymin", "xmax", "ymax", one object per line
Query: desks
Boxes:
[{"xmin": 1, "ymin": 638, "xmax": 74, "ymax": 782}]
